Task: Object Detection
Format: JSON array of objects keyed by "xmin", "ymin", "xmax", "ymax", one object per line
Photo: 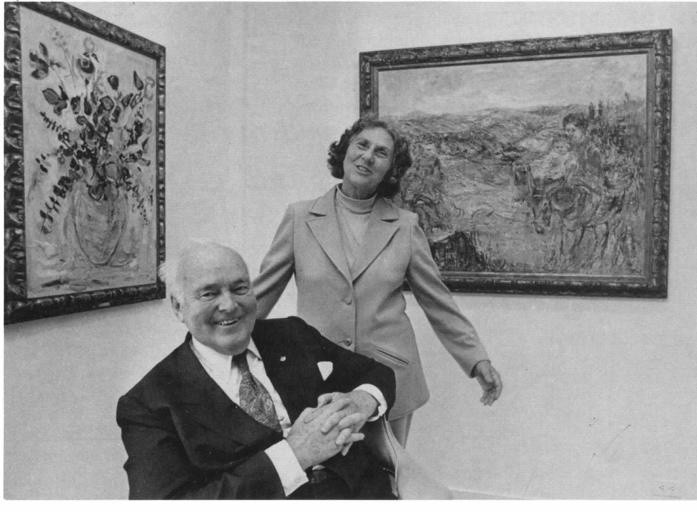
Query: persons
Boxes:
[
  {"xmin": 532, "ymin": 111, "xmax": 608, "ymax": 269},
  {"xmin": 111, "ymin": 238, "xmax": 396, "ymax": 500},
  {"xmin": 539, "ymin": 136, "xmax": 575, "ymax": 182},
  {"xmin": 252, "ymin": 113, "xmax": 501, "ymax": 449}
]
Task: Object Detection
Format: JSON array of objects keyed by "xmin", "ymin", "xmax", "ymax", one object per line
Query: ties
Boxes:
[{"xmin": 231, "ymin": 350, "xmax": 284, "ymax": 439}]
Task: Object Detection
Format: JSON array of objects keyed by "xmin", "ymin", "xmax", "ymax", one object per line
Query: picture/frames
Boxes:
[
  {"xmin": 359, "ymin": 29, "xmax": 672, "ymax": 299},
  {"xmin": 4, "ymin": 2, "xmax": 166, "ymax": 326}
]
